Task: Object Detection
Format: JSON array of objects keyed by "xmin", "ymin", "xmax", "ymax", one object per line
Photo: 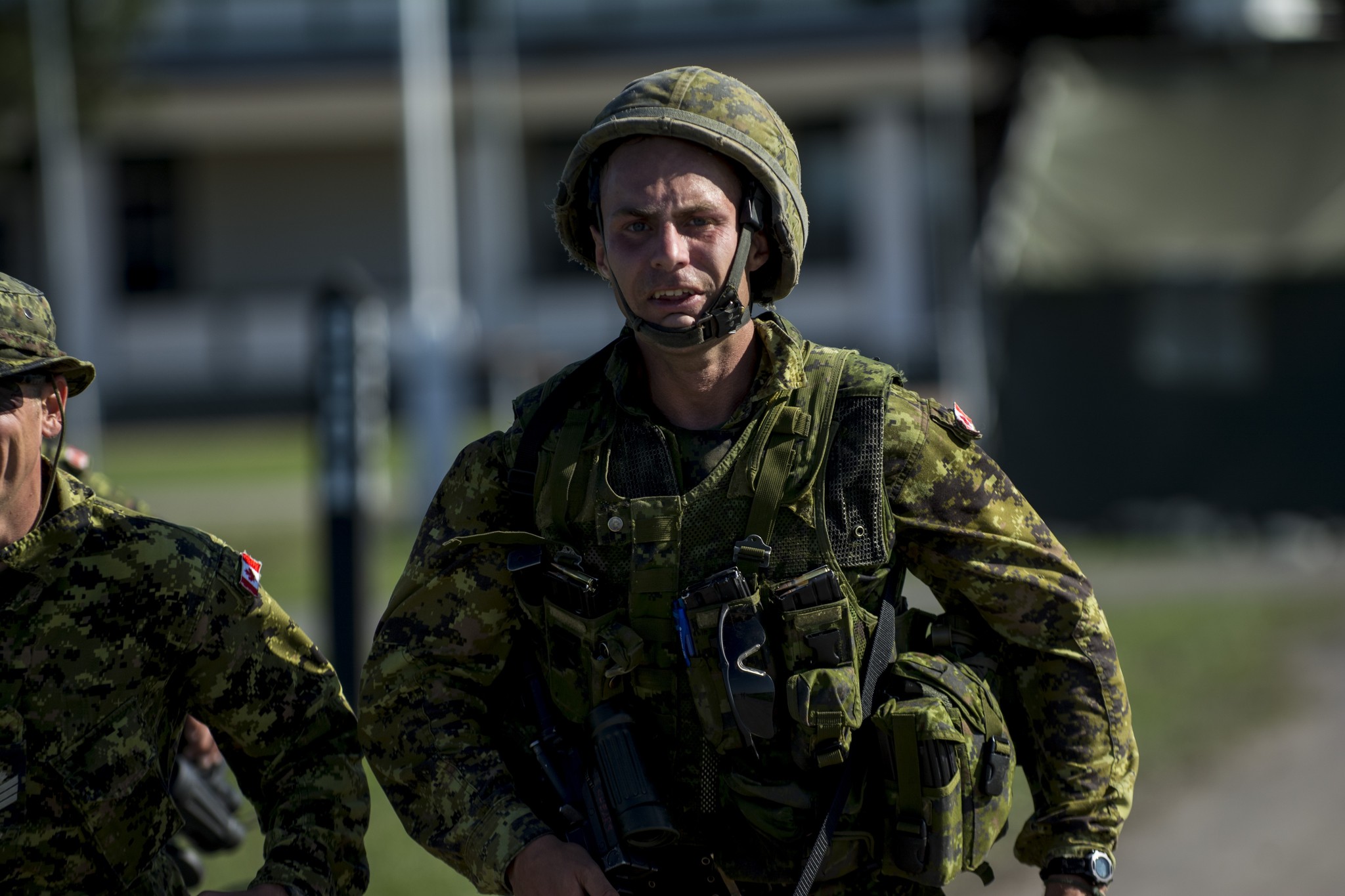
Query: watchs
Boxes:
[{"xmin": 1040, "ymin": 851, "xmax": 1114, "ymax": 885}]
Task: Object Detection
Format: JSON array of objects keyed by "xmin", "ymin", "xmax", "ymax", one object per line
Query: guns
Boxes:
[
  {"xmin": 513, "ymin": 654, "xmax": 682, "ymax": 896},
  {"xmin": 168, "ymin": 730, "xmax": 250, "ymax": 889}
]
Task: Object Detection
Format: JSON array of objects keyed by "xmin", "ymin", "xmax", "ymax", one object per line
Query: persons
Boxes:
[
  {"xmin": 361, "ymin": 66, "xmax": 1141, "ymax": 896},
  {"xmin": 0, "ymin": 274, "xmax": 370, "ymax": 895}
]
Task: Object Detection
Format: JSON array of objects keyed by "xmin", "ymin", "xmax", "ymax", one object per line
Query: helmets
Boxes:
[{"xmin": 554, "ymin": 68, "xmax": 809, "ymax": 303}]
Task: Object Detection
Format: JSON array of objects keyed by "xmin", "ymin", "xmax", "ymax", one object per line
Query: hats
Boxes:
[{"xmin": 0, "ymin": 271, "xmax": 95, "ymax": 400}]
